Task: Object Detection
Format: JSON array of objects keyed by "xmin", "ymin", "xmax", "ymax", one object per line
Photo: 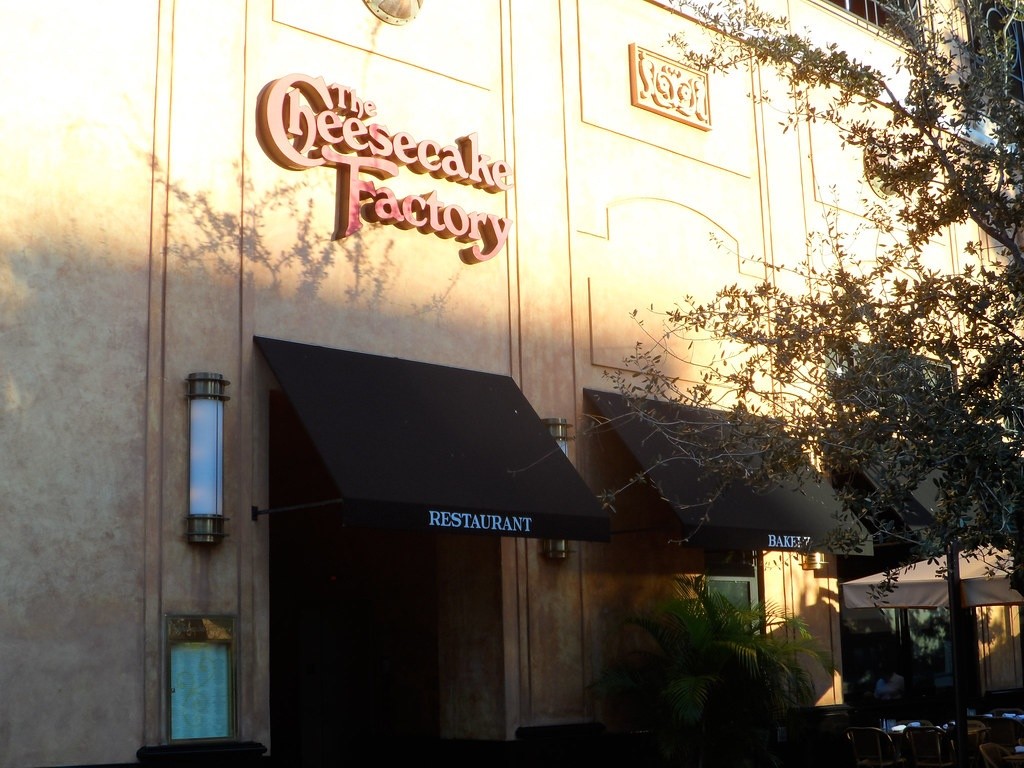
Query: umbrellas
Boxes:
[{"xmin": 841, "ymin": 545, "xmax": 1024, "ymax": 608}]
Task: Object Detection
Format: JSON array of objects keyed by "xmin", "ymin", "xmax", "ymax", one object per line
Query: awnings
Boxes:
[
  {"xmin": 825, "ymin": 430, "xmax": 1024, "ymax": 542},
  {"xmin": 583, "ymin": 388, "xmax": 875, "ymax": 557},
  {"xmin": 253, "ymin": 335, "xmax": 610, "ymax": 543}
]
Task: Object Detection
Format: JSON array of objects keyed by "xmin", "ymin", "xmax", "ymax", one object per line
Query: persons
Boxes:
[{"xmin": 874, "ymin": 662, "xmax": 904, "ymax": 701}]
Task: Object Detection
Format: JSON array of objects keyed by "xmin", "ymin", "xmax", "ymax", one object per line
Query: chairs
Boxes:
[{"xmin": 844, "ymin": 708, "xmax": 1024, "ymax": 768}]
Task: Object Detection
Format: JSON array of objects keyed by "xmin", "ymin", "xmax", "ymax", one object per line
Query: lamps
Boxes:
[
  {"xmin": 539, "ymin": 416, "xmax": 577, "ymax": 560},
  {"xmin": 799, "ymin": 443, "xmax": 829, "ymax": 570},
  {"xmin": 184, "ymin": 372, "xmax": 232, "ymax": 544}
]
